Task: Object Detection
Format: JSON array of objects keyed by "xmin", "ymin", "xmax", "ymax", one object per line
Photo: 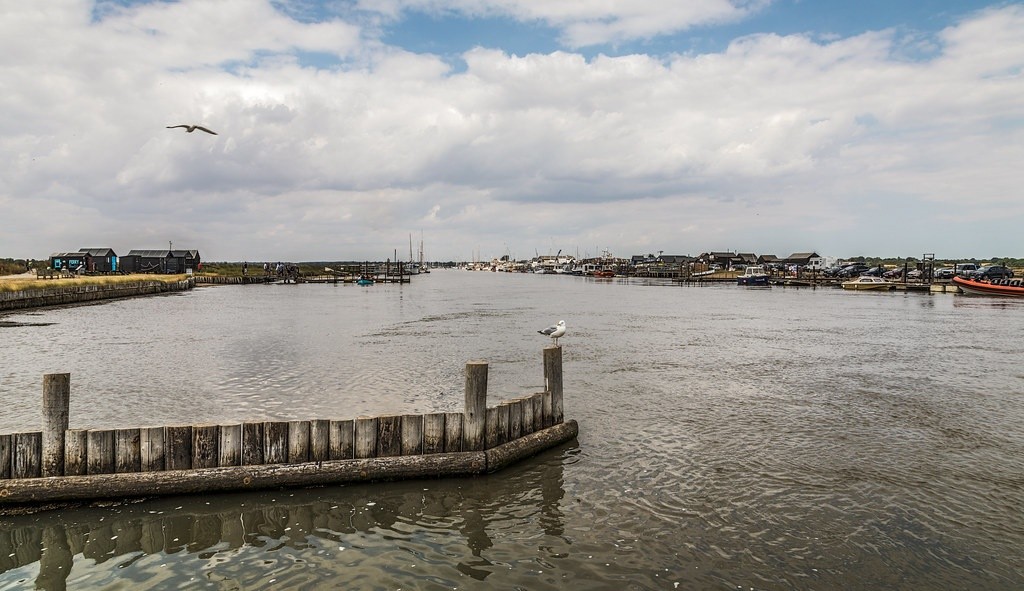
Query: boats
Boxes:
[
  {"xmin": 401, "ymin": 264, "xmax": 420, "ymax": 275},
  {"xmin": 357, "ymin": 279, "xmax": 374, "ymax": 285},
  {"xmin": 953, "ymin": 275, "xmax": 1024, "ymax": 298},
  {"xmin": 572, "ymin": 270, "xmax": 583, "ymax": 276},
  {"xmin": 737, "ymin": 267, "xmax": 771, "ymax": 286},
  {"xmin": 840, "ymin": 276, "xmax": 894, "ymax": 291},
  {"xmin": 783, "ymin": 279, "xmax": 811, "ymax": 287},
  {"xmin": 525, "ymin": 269, "xmax": 534, "ymax": 273}
]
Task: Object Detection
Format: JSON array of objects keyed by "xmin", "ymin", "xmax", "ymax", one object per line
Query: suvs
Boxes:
[{"xmin": 941, "ymin": 262, "xmax": 978, "ymax": 279}]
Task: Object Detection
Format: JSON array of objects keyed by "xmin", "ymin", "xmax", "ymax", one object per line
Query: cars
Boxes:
[
  {"xmin": 708, "ymin": 264, "xmax": 723, "ymax": 271},
  {"xmin": 535, "ymin": 268, "xmax": 544, "ymax": 274},
  {"xmin": 824, "ymin": 261, "xmax": 947, "ymax": 279},
  {"xmin": 786, "ymin": 263, "xmax": 797, "ymax": 270},
  {"xmin": 599, "ymin": 270, "xmax": 615, "ymax": 278},
  {"xmin": 970, "ymin": 265, "xmax": 1014, "ymax": 280}
]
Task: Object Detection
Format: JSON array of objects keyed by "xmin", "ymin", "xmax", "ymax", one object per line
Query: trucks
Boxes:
[{"xmin": 801, "ymin": 258, "xmax": 836, "ymax": 273}]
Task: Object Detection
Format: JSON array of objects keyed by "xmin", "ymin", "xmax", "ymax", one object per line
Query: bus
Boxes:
[
  {"xmin": 543, "ymin": 265, "xmax": 557, "ymax": 274},
  {"xmin": 553, "ymin": 265, "xmax": 576, "ymax": 275}
]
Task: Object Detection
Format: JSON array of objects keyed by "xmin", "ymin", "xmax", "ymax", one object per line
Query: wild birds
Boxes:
[
  {"xmin": 537, "ymin": 320, "xmax": 566, "ymax": 347},
  {"xmin": 166, "ymin": 125, "xmax": 218, "ymax": 136}
]
